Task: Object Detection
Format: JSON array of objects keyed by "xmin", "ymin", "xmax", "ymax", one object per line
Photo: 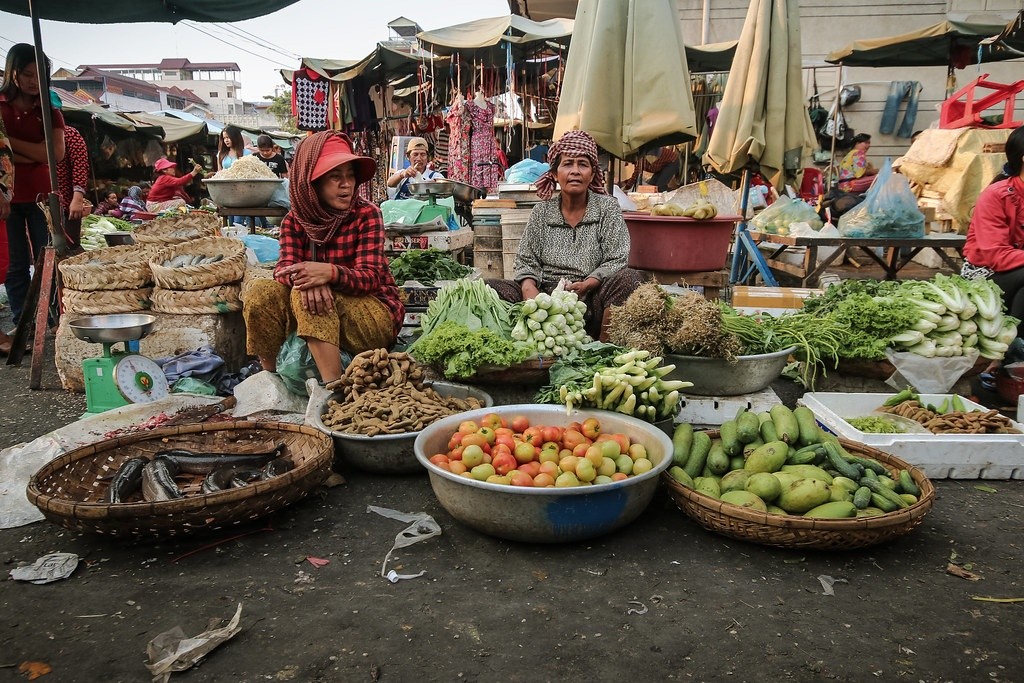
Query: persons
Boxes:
[
  {"xmin": 624, "ymin": 148, "xmax": 679, "ymax": 192},
  {"xmin": 216, "ymin": 125, "xmax": 289, "ymax": 229},
  {"xmin": 839, "ymin": 134, "xmax": 879, "ymax": 194},
  {"xmin": 242, "ymin": 131, "xmax": 406, "ymax": 385},
  {"xmin": 482, "ymin": 131, "xmax": 645, "ymax": 343},
  {"xmin": 961, "ymin": 125, "xmax": 1024, "ymax": 404},
  {"xmin": 387, "ymin": 137, "xmax": 444, "ymax": 203},
  {"xmin": 0, "ymin": 43, "xmax": 64, "ymax": 354},
  {"xmin": 94, "ymin": 158, "xmax": 202, "ymax": 222},
  {"xmin": 51, "ymin": 90, "xmax": 89, "ymax": 253}
]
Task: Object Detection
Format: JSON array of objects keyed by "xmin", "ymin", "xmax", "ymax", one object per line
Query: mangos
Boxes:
[{"xmin": 670, "ymin": 441, "xmax": 857, "ymax": 518}]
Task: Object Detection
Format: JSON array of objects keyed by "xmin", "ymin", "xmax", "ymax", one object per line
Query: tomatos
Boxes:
[{"xmin": 429, "ymin": 413, "xmax": 652, "ymax": 488}]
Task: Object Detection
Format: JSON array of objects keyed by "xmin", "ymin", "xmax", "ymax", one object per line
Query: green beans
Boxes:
[{"xmin": 883, "ymin": 387, "xmax": 980, "ymax": 414}]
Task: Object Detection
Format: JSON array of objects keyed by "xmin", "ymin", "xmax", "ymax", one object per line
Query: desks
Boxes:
[
  {"xmin": 636, "ymin": 270, "xmax": 728, "ymax": 303},
  {"xmin": 386, "ymin": 246, "xmax": 467, "ymax": 265},
  {"xmin": 747, "ymin": 228, "xmax": 969, "ymax": 289},
  {"xmin": 219, "ymin": 207, "xmax": 289, "ymax": 234},
  {"xmin": 499, "ymin": 182, "xmax": 562, "ymax": 203}
]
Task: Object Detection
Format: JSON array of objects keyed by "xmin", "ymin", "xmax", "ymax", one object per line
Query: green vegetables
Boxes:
[
  {"xmin": 80, "ymin": 214, "xmax": 133, "ymax": 231},
  {"xmin": 386, "ymin": 246, "xmax": 473, "ymax": 285},
  {"xmin": 885, "ymin": 273, "xmax": 1022, "ymax": 360},
  {"xmin": 605, "ymin": 279, "xmax": 898, "ymax": 392},
  {"xmin": 404, "ymin": 279, "xmax": 695, "ymax": 425}
]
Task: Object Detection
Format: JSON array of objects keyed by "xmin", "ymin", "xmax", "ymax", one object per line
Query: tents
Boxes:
[{"xmin": 824, "ymin": 20, "xmax": 1024, "ymax": 193}]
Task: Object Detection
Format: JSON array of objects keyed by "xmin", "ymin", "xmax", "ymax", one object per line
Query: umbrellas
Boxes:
[
  {"xmin": 552, "ymin": 0, "xmax": 697, "ymax": 194},
  {"xmin": 701, "ymin": 0, "xmax": 821, "ymax": 283},
  {"xmin": 0, "ymin": 0, "xmax": 298, "ymax": 255}
]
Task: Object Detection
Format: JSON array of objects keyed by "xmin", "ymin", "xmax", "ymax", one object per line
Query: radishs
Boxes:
[{"xmin": 79, "ymin": 224, "xmax": 111, "ymax": 250}]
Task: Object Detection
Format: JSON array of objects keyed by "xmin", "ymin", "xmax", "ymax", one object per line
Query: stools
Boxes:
[
  {"xmin": 938, "ymin": 73, "xmax": 1024, "ymax": 130},
  {"xmin": 388, "ymin": 135, "xmax": 412, "ymax": 176}
]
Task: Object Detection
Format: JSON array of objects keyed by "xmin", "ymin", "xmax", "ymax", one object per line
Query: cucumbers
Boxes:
[{"xmin": 792, "ymin": 441, "xmax": 921, "ymax": 512}]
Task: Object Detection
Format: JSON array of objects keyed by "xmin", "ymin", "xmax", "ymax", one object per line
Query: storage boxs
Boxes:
[
  {"xmin": 761, "ymin": 244, "xmax": 846, "ymax": 267},
  {"xmin": 902, "ymin": 185, "xmax": 961, "ymax": 269},
  {"xmin": 797, "ymin": 392, "xmax": 1024, "ymax": 481},
  {"xmin": 732, "ymin": 285, "xmax": 825, "ymax": 318},
  {"xmin": 381, "ymin": 229, "xmax": 473, "ymax": 251}
]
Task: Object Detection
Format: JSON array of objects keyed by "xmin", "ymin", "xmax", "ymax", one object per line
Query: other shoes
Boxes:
[{"xmin": 6, "ymin": 327, "xmax": 35, "ymax": 339}]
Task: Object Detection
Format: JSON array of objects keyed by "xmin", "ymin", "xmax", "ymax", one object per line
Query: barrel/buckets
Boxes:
[
  {"xmin": 500, "ymin": 209, "xmax": 532, "ymax": 279},
  {"xmin": 471, "ymin": 199, "xmax": 516, "ymax": 281}
]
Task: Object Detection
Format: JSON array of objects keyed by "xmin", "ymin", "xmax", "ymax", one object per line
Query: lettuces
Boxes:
[{"xmin": 793, "ymin": 274, "xmax": 922, "ymax": 359}]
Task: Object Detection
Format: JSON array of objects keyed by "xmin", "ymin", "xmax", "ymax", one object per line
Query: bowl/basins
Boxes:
[
  {"xmin": 103, "ymin": 232, "xmax": 135, "ymax": 246},
  {"xmin": 621, "ymin": 212, "xmax": 744, "ymax": 270},
  {"xmin": 660, "ymin": 346, "xmax": 799, "ymax": 395},
  {"xmin": 315, "ymin": 385, "xmax": 494, "ymax": 470},
  {"xmin": 201, "ymin": 177, "xmax": 284, "ymax": 208},
  {"xmin": 414, "ymin": 403, "xmax": 674, "ymax": 543}
]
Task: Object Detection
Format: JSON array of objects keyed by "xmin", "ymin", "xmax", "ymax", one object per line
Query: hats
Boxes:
[
  {"xmin": 310, "ymin": 134, "xmax": 377, "ymax": 185},
  {"xmin": 407, "ymin": 137, "xmax": 428, "ymax": 152},
  {"xmin": 153, "ymin": 158, "xmax": 177, "ymax": 172},
  {"xmin": 49, "ymin": 90, "xmax": 62, "ymax": 110}
]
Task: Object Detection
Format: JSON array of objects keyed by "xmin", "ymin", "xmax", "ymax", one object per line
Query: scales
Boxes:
[
  {"xmin": 68, "ymin": 314, "xmax": 169, "ymax": 413},
  {"xmin": 406, "ymin": 182, "xmax": 455, "ymax": 224}
]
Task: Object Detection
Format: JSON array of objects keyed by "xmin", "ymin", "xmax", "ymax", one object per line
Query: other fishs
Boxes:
[
  {"xmin": 161, "ymin": 251, "xmax": 224, "ymax": 269},
  {"xmin": 143, "ymin": 456, "xmax": 181, "ymax": 503},
  {"xmin": 154, "ymin": 442, "xmax": 284, "ymax": 475},
  {"xmin": 197, "ymin": 456, "xmax": 289, "ymax": 495},
  {"xmin": 109, "ymin": 453, "xmax": 150, "ymax": 505}
]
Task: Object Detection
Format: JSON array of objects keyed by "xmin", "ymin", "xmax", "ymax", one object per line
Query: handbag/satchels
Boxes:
[
  {"xmin": 807, "ymin": 93, "xmax": 845, "ymax": 140},
  {"xmin": 841, "ymin": 156, "xmax": 925, "ymax": 255},
  {"xmin": 755, "ymin": 193, "xmax": 823, "ymax": 238}
]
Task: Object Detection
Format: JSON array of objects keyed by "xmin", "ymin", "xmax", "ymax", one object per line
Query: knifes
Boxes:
[{"xmin": 166, "ymin": 396, "xmax": 236, "ymax": 425}]
